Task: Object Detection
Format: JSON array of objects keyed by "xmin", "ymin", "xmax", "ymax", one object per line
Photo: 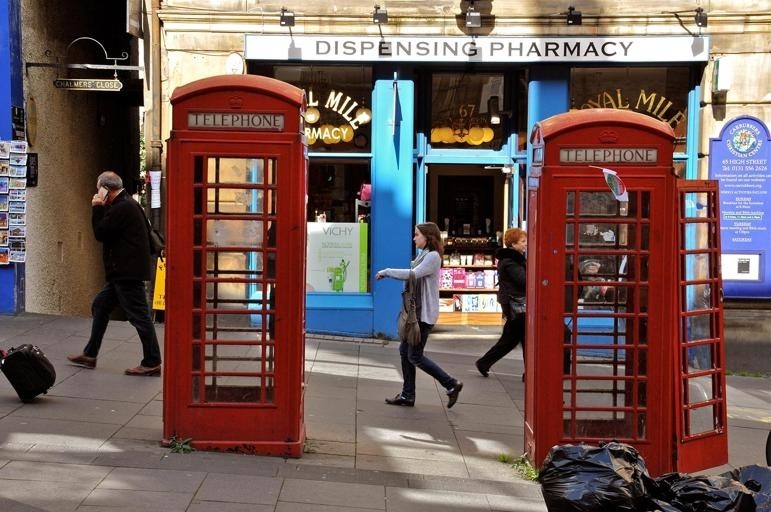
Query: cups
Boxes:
[
  {"xmin": 315, "ymin": 216, "xmax": 326, "ymax": 223},
  {"xmin": 460, "ymin": 255, "xmax": 473, "ymax": 265}
]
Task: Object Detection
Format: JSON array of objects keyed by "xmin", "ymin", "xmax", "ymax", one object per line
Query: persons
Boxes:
[
  {"xmin": 475, "ymin": 228, "xmax": 527, "ymax": 382},
  {"xmin": 66, "ymin": 171, "xmax": 162, "ymax": 377},
  {"xmin": 374, "ymin": 222, "xmax": 464, "ymax": 409}
]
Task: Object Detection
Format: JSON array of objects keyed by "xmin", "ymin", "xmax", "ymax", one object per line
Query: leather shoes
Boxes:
[
  {"xmin": 446, "ymin": 380, "xmax": 463, "ymax": 408},
  {"xmin": 385, "ymin": 394, "xmax": 415, "ymax": 406}
]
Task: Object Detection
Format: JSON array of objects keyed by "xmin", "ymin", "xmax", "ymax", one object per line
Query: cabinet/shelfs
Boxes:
[{"xmin": 436, "ymin": 238, "xmax": 506, "ymax": 325}]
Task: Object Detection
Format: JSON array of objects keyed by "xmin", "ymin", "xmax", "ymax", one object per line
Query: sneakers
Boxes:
[
  {"xmin": 474, "ymin": 361, "xmax": 488, "ymax": 377},
  {"xmin": 67, "ymin": 353, "xmax": 97, "ymax": 369},
  {"xmin": 125, "ymin": 364, "xmax": 161, "ymax": 377}
]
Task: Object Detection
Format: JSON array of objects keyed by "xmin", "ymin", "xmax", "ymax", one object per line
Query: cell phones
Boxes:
[{"xmin": 98, "ymin": 185, "xmax": 109, "ymax": 201}]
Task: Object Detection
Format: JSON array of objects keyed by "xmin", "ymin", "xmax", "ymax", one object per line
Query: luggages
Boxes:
[{"xmin": 0, "ymin": 344, "xmax": 56, "ymax": 405}]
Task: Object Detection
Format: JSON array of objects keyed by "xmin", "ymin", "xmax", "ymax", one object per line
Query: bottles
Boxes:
[
  {"xmin": 467, "ymin": 270, "xmax": 484, "ymax": 288},
  {"xmin": 484, "ymin": 255, "xmax": 493, "ymax": 266}
]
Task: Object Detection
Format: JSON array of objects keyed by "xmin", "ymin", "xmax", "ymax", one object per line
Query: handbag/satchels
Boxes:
[
  {"xmin": 148, "ymin": 228, "xmax": 166, "ymax": 256},
  {"xmin": 509, "ymin": 295, "xmax": 526, "ymax": 321},
  {"xmin": 398, "ymin": 291, "xmax": 422, "ymax": 347}
]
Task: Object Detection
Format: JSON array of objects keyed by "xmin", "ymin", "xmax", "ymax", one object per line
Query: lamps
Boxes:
[
  {"xmin": 465, "ymin": 6, "xmax": 481, "ymax": 28},
  {"xmin": 662, "ymin": 7, "xmax": 707, "ymax": 27},
  {"xmin": 373, "ymin": 4, "xmax": 388, "ymax": 24},
  {"xmin": 567, "ymin": 6, "xmax": 582, "ymax": 25},
  {"xmin": 279, "ymin": 7, "xmax": 295, "ymax": 26}
]
